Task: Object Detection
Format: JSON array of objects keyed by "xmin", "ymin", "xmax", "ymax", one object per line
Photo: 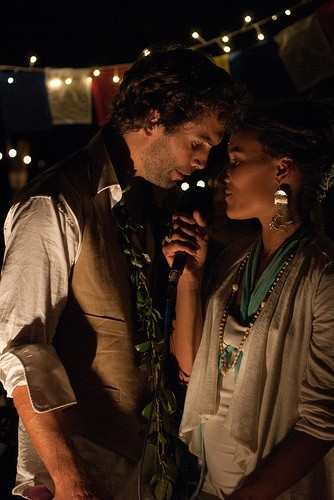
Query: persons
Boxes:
[
  {"xmin": 160, "ymin": 98, "xmax": 334, "ymax": 500},
  {"xmin": 1, "ymin": 41, "xmax": 254, "ymax": 500}
]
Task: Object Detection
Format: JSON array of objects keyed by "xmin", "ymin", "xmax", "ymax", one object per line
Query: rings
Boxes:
[{"xmin": 164, "ymin": 233, "xmax": 173, "ymax": 244}]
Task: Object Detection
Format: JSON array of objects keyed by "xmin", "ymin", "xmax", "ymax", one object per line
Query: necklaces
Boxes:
[{"xmin": 217, "ymin": 234, "xmax": 311, "ymax": 377}]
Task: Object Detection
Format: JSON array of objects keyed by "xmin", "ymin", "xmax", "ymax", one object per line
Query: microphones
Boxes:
[{"xmin": 169, "ymin": 187, "xmax": 208, "ymax": 284}]
